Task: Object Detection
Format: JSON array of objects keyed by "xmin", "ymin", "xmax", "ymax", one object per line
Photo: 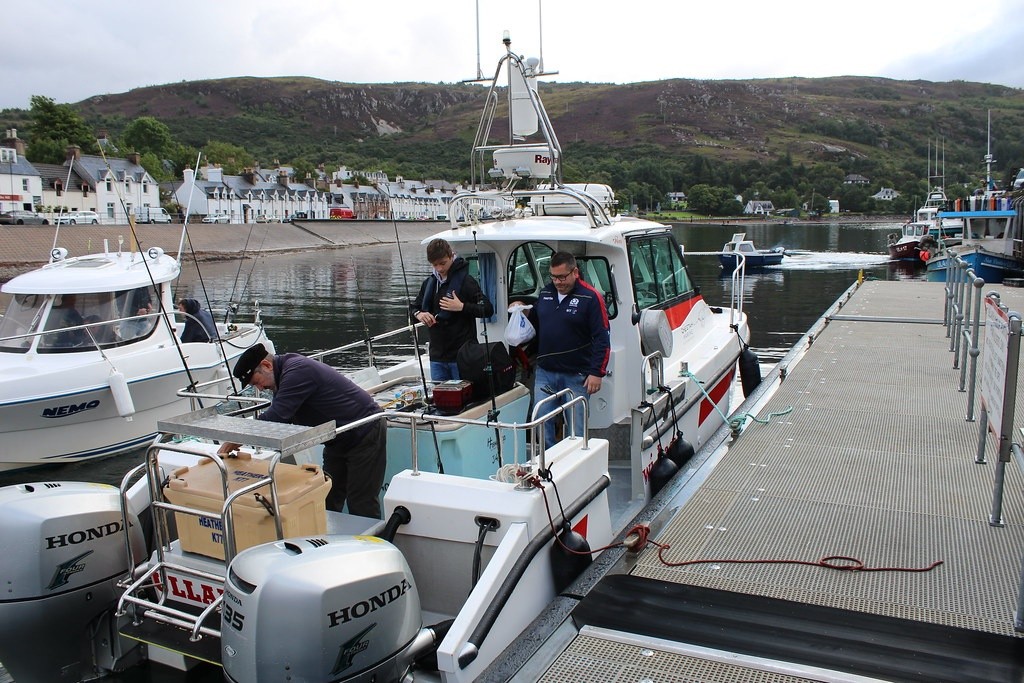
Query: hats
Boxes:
[{"xmin": 233, "ymin": 343, "xmax": 269, "ymax": 390}]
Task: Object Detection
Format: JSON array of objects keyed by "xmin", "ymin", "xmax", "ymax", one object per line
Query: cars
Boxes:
[
  {"xmin": 256, "ymin": 214, "xmax": 281, "ymax": 223},
  {"xmin": 55, "ymin": 211, "xmax": 100, "ymax": 226},
  {"xmin": 0, "ymin": 209, "xmax": 49, "ymax": 226},
  {"xmin": 202, "ymin": 213, "xmax": 230, "ymax": 224},
  {"xmin": 283, "ymin": 211, "xmax": 309, "ymax": 223}
]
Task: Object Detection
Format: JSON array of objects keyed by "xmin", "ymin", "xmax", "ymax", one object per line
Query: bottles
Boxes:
[{"xmin": 395, "ymin": 384, "xmax": 435, "ymax": 399}]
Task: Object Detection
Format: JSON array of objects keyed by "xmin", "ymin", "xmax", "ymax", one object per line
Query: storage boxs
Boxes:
[{"xmin": 159, "ymin": 450, "xmax": 332, "ymax": 560}]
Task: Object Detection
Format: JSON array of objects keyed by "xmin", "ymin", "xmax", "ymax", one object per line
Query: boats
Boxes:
[
  {"xmin": 886, "ymin": 108, "xmax": 1024, "ymax": 283},
  {"xmin": 718, "ymin": 233, "xmax": 792, "ymax": 268},
  {"xmin": 0, "ymin": 154, "xmax": 277, "ymax": 473},
  {"xmin": 0, "ymin": 1, "xmax": 777, "ymax": 683}
]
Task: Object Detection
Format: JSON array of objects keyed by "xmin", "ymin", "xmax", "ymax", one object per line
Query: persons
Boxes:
[
  {"xmin": 177, "ymin": 205, "xmax": 190, "ymax": 225},
  {"xmin": 178, "ymin": 298, "xmax": 218, "ymax": 343},
  {"xmin": 409, "ymin": 239, "xmax": 494, "ymax": 385},
  {"xmin": 115, "ymin": 286, "xmax": 153, "ymax": 340},
  {"xmin": 509, "ymin": 251, "xmax": 611, "ymax": 451},
  {"xmin": 51, "ymin": 308, "xmax": 123, "ymax": 347},
  {"xmin": 217, "ymin": 343, "xmax": 388, "ymax": 520}
]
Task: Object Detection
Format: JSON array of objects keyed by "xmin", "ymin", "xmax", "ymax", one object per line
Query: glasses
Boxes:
[{"xmin": 549, "ymin": 269, "xmax": 573, "ymax": 281}]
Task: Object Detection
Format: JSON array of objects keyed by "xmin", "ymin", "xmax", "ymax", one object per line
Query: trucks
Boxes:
[
  {"xmin": 331, "ymin": 209, "xmax": 357, "ymax": 219},
  {"xmin": 134, "ymin": 207, "xmax": 172, "ymax": 224}
]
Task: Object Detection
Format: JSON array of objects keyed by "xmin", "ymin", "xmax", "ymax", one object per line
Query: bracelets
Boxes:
[{"xmin": 414, "ymin": 311, "xmax": 420, "ymax": 316}]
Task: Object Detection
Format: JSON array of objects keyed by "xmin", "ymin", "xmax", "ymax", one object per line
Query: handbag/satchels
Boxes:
[
  {"xmin": 457, "ymin": 338, "xmax": 513, "ymax": 396},
  {"xmin": 504, "ymin": 304, "xmax": 536, "ymax": 347}
]
